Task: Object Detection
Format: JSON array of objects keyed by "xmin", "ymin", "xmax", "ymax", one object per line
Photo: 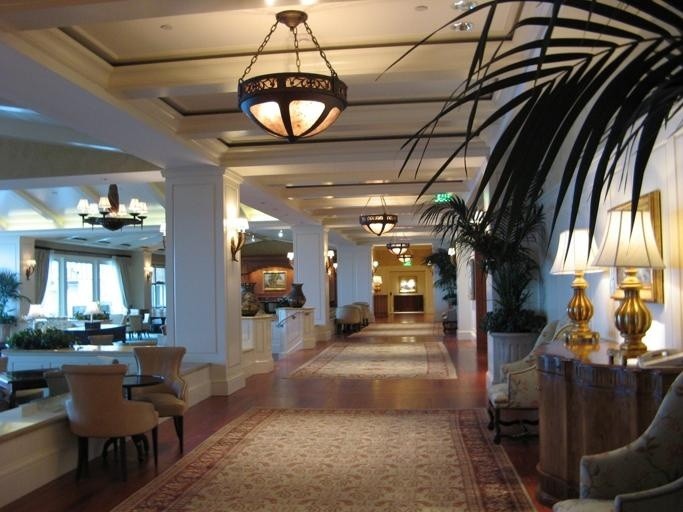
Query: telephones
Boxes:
[{"xmin": 639, "ymin": 349, "xmax": 683, "ymax": 368}]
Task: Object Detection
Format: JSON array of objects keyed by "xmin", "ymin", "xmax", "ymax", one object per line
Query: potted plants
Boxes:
[
  {"xmin": 407, "ymin": 180, "xmax": 550, "ymax": 404},
  {"xmin": 416, "ymin": 245, "xmax": 457, "ymax": 336}
]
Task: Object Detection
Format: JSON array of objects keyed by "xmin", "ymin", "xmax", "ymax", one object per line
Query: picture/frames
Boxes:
[
  {"xmin": 604, "ymin": 189, "xmax": 665, "ymax": 305},
  {"xmin": 262, "ymin": 270, "xmax": 288, "ymax": 292}
]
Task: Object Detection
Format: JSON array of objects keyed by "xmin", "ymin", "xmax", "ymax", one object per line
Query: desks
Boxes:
[{"xmin": 531, "ymin": 335, "xmax": 683, "ymax": 508}]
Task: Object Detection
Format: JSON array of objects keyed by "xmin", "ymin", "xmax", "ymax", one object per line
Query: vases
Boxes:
[
  {"xmin": 240, "ymin": 281, "xmax": 262, "ymax": 317},
  {"xmin": 285, "ymin": 282, "xmax": 307, "ymax": 308}
]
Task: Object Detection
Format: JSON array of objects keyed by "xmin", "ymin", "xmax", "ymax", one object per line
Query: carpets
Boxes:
[
  {"xmin": 346, "ymin": 322, "xmax": 447, "ymax": 338},
  {"xmin": 106, "ymin": 405, "xmax": 538, "ymax": 511},
  {"xmin": 288, "ymin": 339, "xmax": 459, "ymax": 380}
]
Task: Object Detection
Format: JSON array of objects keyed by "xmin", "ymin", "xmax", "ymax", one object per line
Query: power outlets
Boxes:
[{"xmin": 385, "ymin": 224, "xmax": 410, "ymax": 255}]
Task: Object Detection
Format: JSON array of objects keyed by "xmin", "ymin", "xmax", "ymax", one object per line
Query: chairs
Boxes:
[
  {"xmin": 0, "ymin": 303, "xmax": 189, "ymax": 484},
  {"xmin": 549, "ymin": 367, "xmax": 682, "ymax": 511},
  {"xmin": 334, "ymin": 301, "xmax": 370, "ymax": 337},
  {"xmin": 485, "ymin": 317, "xmax": 575, "ymax": 445}
]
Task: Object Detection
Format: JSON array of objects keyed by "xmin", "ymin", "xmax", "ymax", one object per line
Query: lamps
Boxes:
[
  {"xmin": 359, "ymin": 194, "xmax": 398, "ymax": 238},
  {"xmin": 223, "ymin": 212, "xmax": 250, "ymax": 263},
  {"xmin": 236, "ymin": 8, "xmax": 349, "ymax": 147},
  {"xmin": 549, "ymin": 226, "xmax": 607, "ymax": 353},
  {"xmin": 589, "ymin": 208, "xmax": 668, "ymax": 360},
  {"xmin": 75, "ymin": 184, "xmax": 149, "ymax": 233}
]
